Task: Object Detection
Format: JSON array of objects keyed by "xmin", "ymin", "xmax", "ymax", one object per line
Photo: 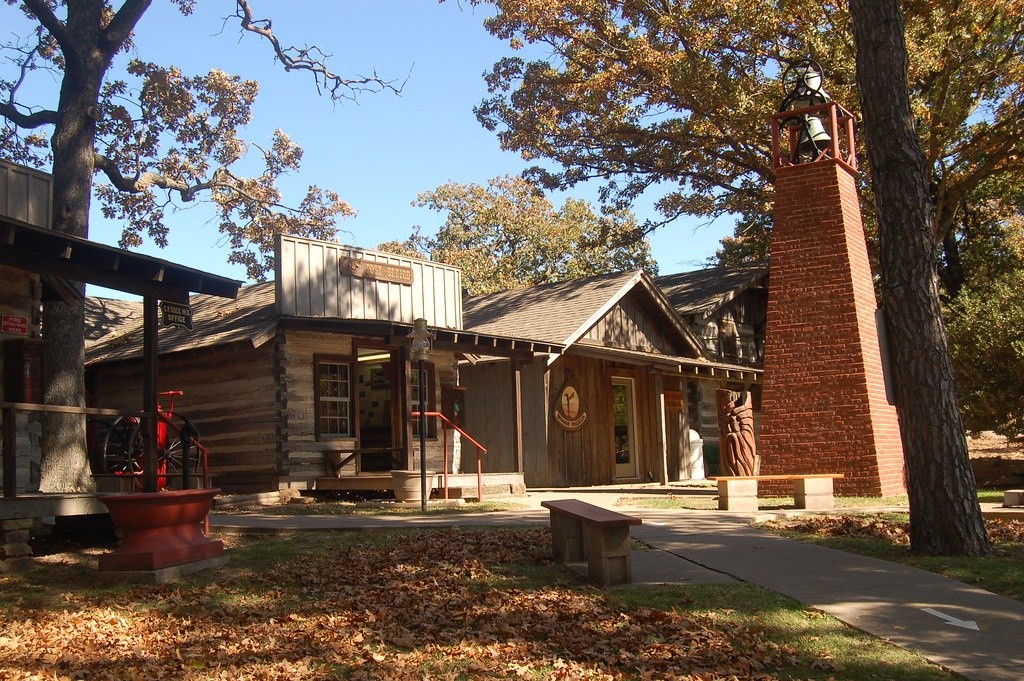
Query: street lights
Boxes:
[{"xmin": 406, "ymin": 317, "xmax": 436, "ymax": 513}]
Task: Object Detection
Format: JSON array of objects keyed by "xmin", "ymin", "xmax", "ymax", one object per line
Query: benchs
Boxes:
[
  {"xmin": 707, "ymin": 473, "xmax": 844, "ymax": 512},
  {"xmin": 542, "ymin": 499, "xmax": 642, "ymax": 586}
]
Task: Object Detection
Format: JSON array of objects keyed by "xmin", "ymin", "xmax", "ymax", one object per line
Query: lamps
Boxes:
[{"xmin": 404, "ymin": 317, "xmax": 434, "ymax": 362}]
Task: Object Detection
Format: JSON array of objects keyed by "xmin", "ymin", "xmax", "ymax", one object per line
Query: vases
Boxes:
[{"xmin": 391, "ymin": 469, "xmax": 436, "ymax": 502}]
